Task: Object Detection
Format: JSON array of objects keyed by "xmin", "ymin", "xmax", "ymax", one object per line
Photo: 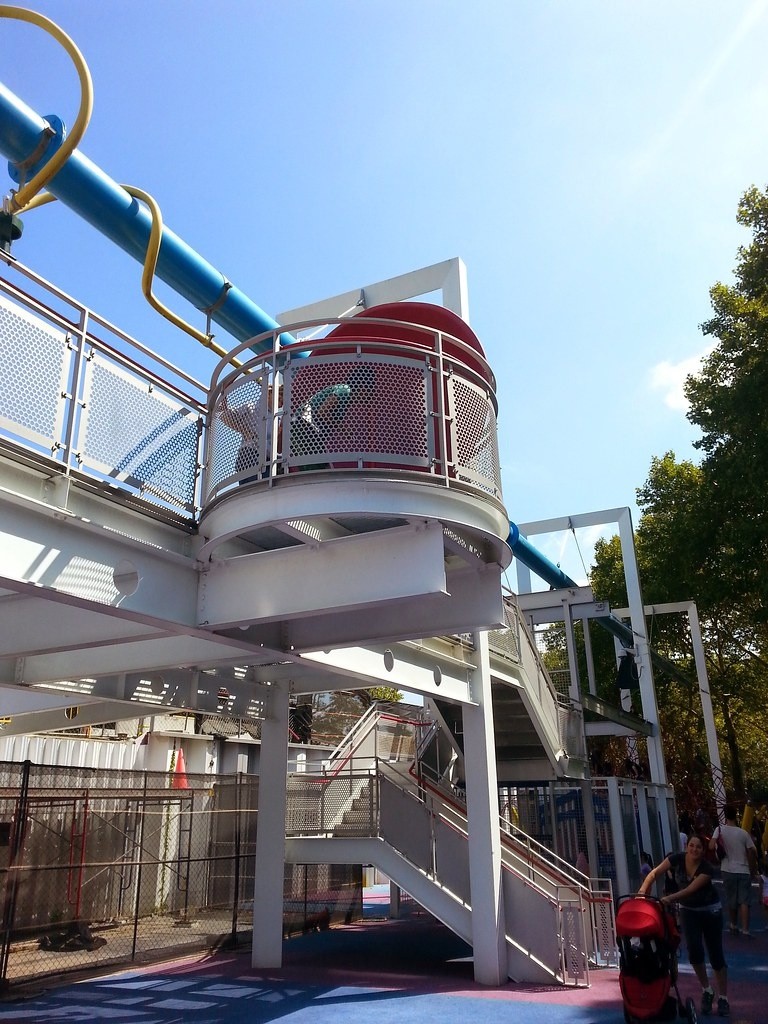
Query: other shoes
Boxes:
[
  {"xmin": 727, "ymin": 921, "xmax": 739, "ymax": 932},
  {"xmin": 743, "ymin": 934, "xmax": 756, "ymax": 940}
]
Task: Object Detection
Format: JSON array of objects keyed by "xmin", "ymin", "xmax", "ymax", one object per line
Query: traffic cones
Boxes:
[{"xmin": 173, "ymin": 747, "xmax": 189, "ymax": 788}]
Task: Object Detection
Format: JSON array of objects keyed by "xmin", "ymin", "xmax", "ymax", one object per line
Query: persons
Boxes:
[
  {"xmin": 293, "ymin": 366, "xmax": 374, "ymax": 470},
  {"xmin": 221, "ymin": 384, "xmax": 283, "ymax": 486},
  {"xmin": 635, "ymin": 802, "xmax": 768, "ymax": 1016},
  {"xmin": 576, "ymin": 840, "xmax": 589, "ymax": 878}
]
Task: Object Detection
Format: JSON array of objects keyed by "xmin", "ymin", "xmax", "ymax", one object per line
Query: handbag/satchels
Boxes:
[{"xmin": 714, "ymin": 826, "xmax": 726, "ymax": 861}]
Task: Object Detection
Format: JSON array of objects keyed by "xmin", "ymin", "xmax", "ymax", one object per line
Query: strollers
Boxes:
[{"xmin": 615, "ymin": 894, "xmax": 698, "ymax": 1024}]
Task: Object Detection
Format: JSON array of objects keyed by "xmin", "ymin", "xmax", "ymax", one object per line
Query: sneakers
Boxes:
[
  {"xmin": 718, "ymin": 998, "xmax": 729, "ymax": 1016},
  {"xmin": 702, "ymin": 991, "xmax": 714, "ymax": 1016}
]
